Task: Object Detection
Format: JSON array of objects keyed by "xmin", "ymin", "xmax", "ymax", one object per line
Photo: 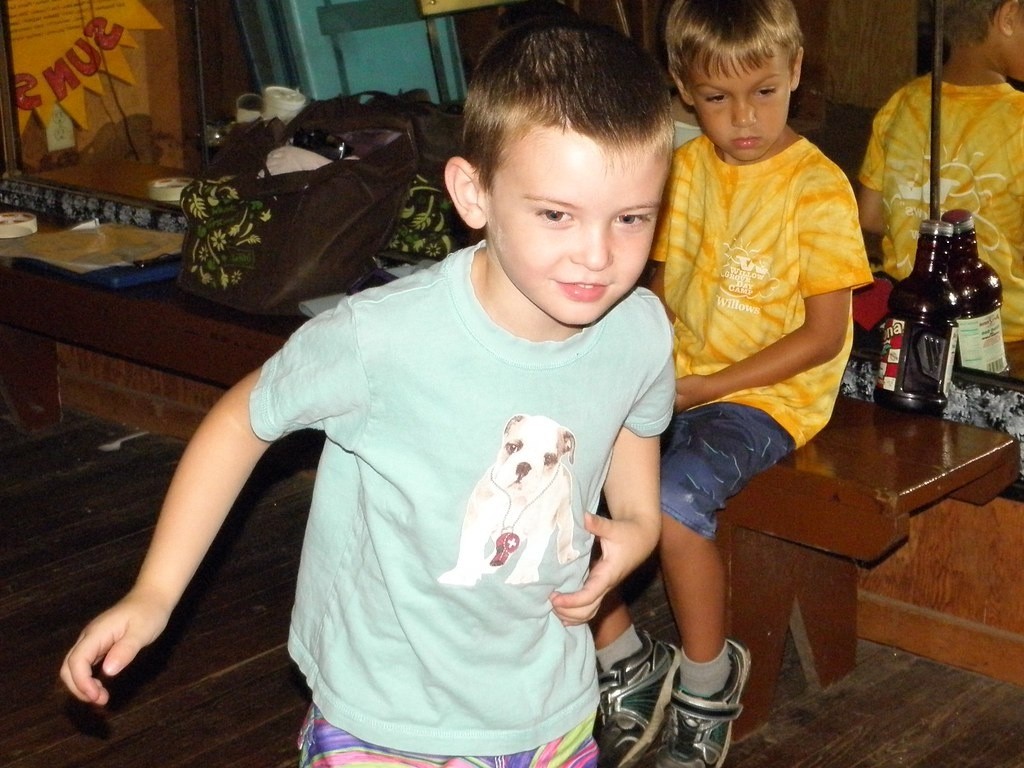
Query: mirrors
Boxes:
[{"xmin": 2, "ymin": 1, "xmax": 1024, "ymax": 392}]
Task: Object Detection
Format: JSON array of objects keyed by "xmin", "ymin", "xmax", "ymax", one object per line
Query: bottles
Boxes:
[
  {"xmin": 942, "ymin": 209, "xmax": 1009, "ymax": 374},
  {"xmin": 874, "ymin": 222, "xmax": 953, "ymax": 415}
]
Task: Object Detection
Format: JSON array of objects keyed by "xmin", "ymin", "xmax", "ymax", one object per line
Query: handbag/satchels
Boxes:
[
  {"xmin": 288, "ymin": 90, "xmax": 468, "ymax": 258},
  {"xmin": 177, "ymin": 115, "xmax": 421, "ymax": 314}
]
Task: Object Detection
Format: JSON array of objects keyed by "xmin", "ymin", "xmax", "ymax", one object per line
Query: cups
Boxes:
[{"xmin": 261, "ymin": 86, "xmax": 305, "ymax": 124}]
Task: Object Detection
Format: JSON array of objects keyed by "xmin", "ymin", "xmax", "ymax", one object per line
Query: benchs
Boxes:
[
  {"xmin": 0, "ymin": 266, "xmax": 1020, "ymax": 743},
  {"xmin": 32, "ymin": 159, "xmax": 1024, "ymax": 381}
]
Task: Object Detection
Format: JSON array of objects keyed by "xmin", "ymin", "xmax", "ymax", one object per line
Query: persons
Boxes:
[
  {"xmin": 588, "ymin": 0, "xmax": 873, "ymax": 768},
  {"xmin": 58, "ymin": 20, "xmax": 678, "ymax": 768},
  {"xmin": 857, "ymin": 2, "xmax": 1024, "ymax": 343}
]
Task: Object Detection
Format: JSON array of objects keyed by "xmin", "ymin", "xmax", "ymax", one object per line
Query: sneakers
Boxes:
[
  {"xmin": 591, "ymin": 628, "xmax": 682, "ymax": 768},
  {"xmin": 656, "ymin": 639, "xmax": 751, "ymax": 768}
]
